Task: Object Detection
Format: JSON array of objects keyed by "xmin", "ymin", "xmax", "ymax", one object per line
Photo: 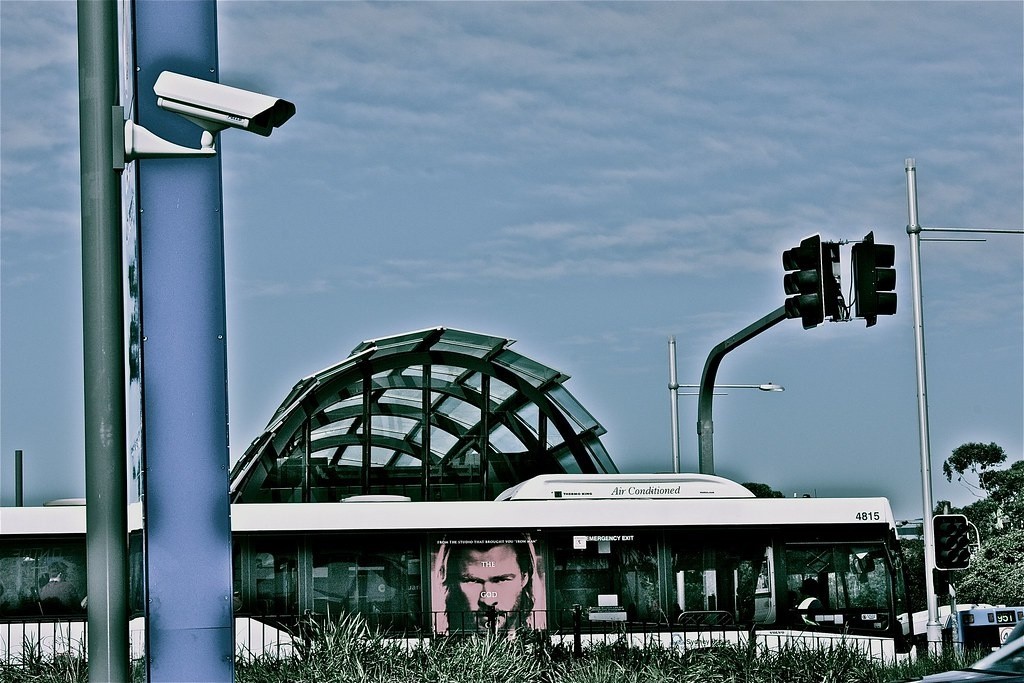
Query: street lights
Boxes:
[{"xmin": 667, "ymin": 335, "xmax": 785, "ymax": 475}]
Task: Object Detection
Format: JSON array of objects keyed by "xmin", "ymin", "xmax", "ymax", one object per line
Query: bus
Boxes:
[
  {"xmin": 1, "ymin": 474, "xmax": 919, "ymax": 672},
  {"xmin": 897, "ymin": 603, "xmax": 1024, "ymax": 662}
]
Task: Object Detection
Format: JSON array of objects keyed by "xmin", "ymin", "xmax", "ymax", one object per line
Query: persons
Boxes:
[
  {"xmin": 39, "ymin": 562, "xmax": 81, "ymax": 611},
  {"xmin": 435, "ymin": 532, "xmax": 537, "ymax": 630}
]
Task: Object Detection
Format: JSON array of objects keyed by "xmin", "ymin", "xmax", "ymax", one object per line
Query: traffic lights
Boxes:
[
  {"xmin": 862, "ymin": 231, "xmax": 898, "ymax": 328},
  {"xmin": 930, "ymin": 514, "xmax": 973, "ymax": 571},
  {"xmin": 782, "ymin": 232, "xmax": 826, "ymax": 331}
]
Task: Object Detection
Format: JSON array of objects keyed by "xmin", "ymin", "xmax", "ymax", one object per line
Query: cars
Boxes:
[{"xmin": 883, "ymin": 617, "xmax": 1024, "ymax": 683}]
